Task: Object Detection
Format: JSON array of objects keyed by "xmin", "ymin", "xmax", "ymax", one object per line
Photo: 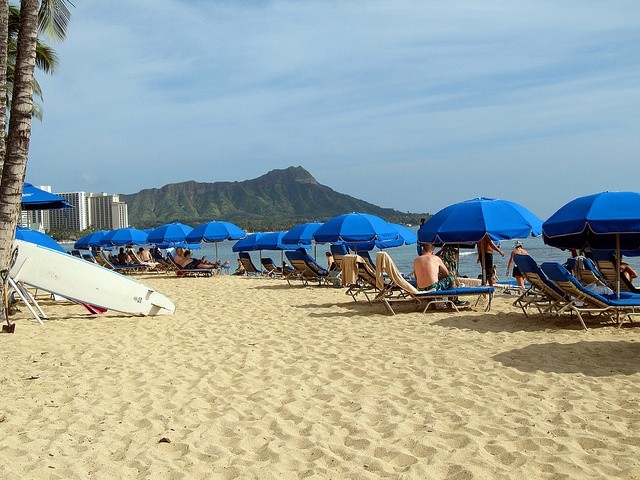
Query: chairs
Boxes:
[
  {"xmin": 259, "ymin": 258, "xmax": 295, "ymax": 279},
  {"xmin": 64, "ymin": 244, "xmax": 218, "ymax": 279},
  {"xmin": 240, "ymin": 252, "xmax": 268, "ymax": 276},
  {"xmin": 341, "ymin": 254, "xmax": 416, "ymax": 304},
  {"xmin": 283, "ymin": 249, "xmax": 331, "ymax": 287},
  {"xmin": 355, "ymin": 250, "xmax": 377, "ymax": 272},
  {"xmin": 304, "ymin": 253, "xmax": 330, "ymax": 277},
  {"xmin": 277, "ymin": 265, "xmax": 299, "ymax": 280},
  {"xmin": 581, "ymin": 247, "xmax": 639, "ymax": 294},
  {"xmin": 565, "ymin": 255, "xmax": 616, "ymax": 297},
  {"xmin": 372, "ymin": 251, "xmax": 494, "ymax": 318},
  {"xmin": 330, "ymin": 243, "xmax": 351, "ymax": 270},
  {"xmin": 509, "ymin": 253, "xmax": 576, "ymax": 321},
  {"xmin": 536, "ymin": 260, "xmax": 640, "ymax": 329}
]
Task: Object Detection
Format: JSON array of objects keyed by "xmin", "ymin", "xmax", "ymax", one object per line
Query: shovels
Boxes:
[{"xmin": 0, "ymin": 269, "xmax": 15, "ymax": 333}]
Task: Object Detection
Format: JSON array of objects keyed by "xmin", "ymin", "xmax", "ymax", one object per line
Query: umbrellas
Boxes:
[
  {"xmin": 416, "ymin": 196, "xmax": 545, "ymax": 300},
  {"xmin": 329, "ymin": 223, "xmax": 418, "ymax": 253},
  {"xmin": 21, "ymin": 201, "xmax": 76, "ymax": 211},
  {"xmin": 312, "ymin": 211, "xmax": 398, "ymax": 255},
  {"xmin": 15, "ymin": 225, "xmax": 66, "ymax": 253},
  {"xmin": 185, "ymin": 220, "xmax": 246, "ymax": 261},
  {"xmin": 154, "ymin": 241, "xmax": 202, "ymax": 251},
  {"xmin": 147, "ymin": 221, "xmax": 193, "ymax": 248},
  {"xmin": 254, "ymin": 231, "xmax": 312, "ymax": 265},
  {"xmin": 541, "ymin": 191, "xmax": 640, "ymax": 324},
  {"xmin": 281, "ymin": 220, "xmax": 326, "ymax": 261},
  {"xmin": 74, "ymin": 231, "xmax": 114, "ymax": 250},
  {"xmin": 232, "ymin": 231, "xmax": 264, "ymax": 276},
  {"xmin": 100, "ymin": 227, "xmax": 148, "ymax": 247},
  {"xmin": 20, "ymin": 181, "xmax": 66, "ymax": 227}
]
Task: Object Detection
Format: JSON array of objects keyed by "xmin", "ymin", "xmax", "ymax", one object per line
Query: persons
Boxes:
[
  {"xmin": 118, "ymin": 248, "xmax": 127, "ymax": 266},
  {"xmin": 326, "ymin": 252, "xmax": 335, "ymax": 271},
  {"xmin": 152, "ymin": 247, "xmax": 162, "ymax": 260},
  {"xmin": 506, "ymin": 241, "xmax": 529, "ymax": 290},
  {"xmin": 619, "ymin": 254, "xmax": 638, "ymax": 287},
  {"xmin": 138, "ymin": 247, "xmax": 152, "ymax": 263},
  {"xmin": 493, "ymin": 264, "xmax": 500, "ymax": 280},
  {"xmin": 174, "ymin": 247, "xmax": 229, "ymax": 269},
  {"xmin": 235, "ymin": 259, "xmax": 245, "ymax": 275},
  {"xmin": 476, "ymin": 235, "xmax": 505, "ymax": 287},
  {"xmin": 412, "ymin": 243, "xmax": 467, "ymax": 306}
]
